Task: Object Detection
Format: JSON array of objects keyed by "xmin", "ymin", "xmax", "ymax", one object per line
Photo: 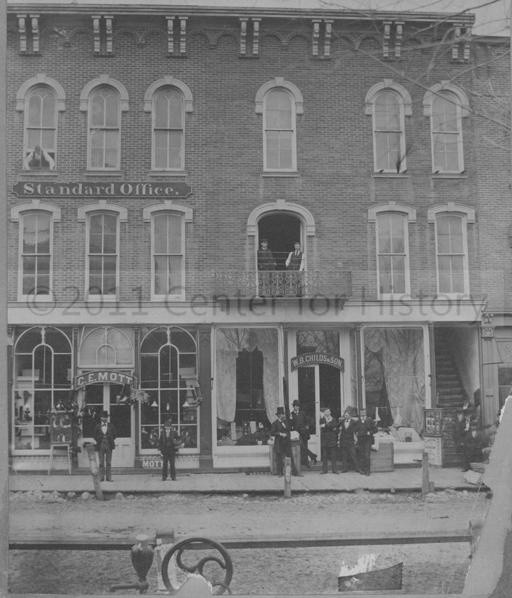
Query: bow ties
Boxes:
[{"xmin": 102, "ymin": 424, "xmax": 107, "ymax": 426}]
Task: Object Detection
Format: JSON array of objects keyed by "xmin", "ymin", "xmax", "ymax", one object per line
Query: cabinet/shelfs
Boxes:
[{"xmin": 423, "ymin": 408, "xmax": 443, "ymax": 467}]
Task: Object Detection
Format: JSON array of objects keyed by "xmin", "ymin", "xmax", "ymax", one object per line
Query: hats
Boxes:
[
  {"xmin": 293, "ymin": 400, "xmax": 301, "ymax": 406},
  {"xmin": 100, "ymin": 411, "xmax": 109, "ymax": 417},
  {"xmin": 164, "ymin": 421, "xmax": 171, "ymax": 428},
  {"xmin": 360, "ymin": 409, "xmax": 367, "ymax": 416},
  {"xmin": 275, "ymin": 407, "xmax": 284, "ymax": 414}
]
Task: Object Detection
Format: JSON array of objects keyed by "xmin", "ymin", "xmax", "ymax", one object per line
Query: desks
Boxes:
[{"xmin": 48, "ymin": 444, "xmax": 72, "ymax": 475}]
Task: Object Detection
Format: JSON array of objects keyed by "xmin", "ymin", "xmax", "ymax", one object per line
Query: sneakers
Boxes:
[
  {"xmin": 99, "ymin": 478, "xmax": 113, "ymax": 482},
  {"xmin": 312, "ymin": 455, "xmax": 317, "ymax": 464},
  {"xmin": 163, "ymin": 475, "xmax": 176, "ymax": 480},
  {"xmin": 302, "ymin": 461, "xmax": 309, "ymax": 465},
  {"xmin": 293, "ymin": 471, "xmax": 298, "ymax": 475},
  {"xmin": 320, "ymin": 468, "xmax": 370, "ymax": 475}
]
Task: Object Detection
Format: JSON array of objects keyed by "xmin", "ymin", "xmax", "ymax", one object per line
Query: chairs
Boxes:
[{"xmin": 370, "ymin": 442, "xmax": 394, "ymax": 472}]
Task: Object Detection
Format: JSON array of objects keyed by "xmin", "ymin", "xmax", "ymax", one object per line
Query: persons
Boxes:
[
  {"xmin": 95, "ymin": 411, "xmax": 116, "ymax": 481},
  {"xmin": 292, "ymin": 400, "xmax": 317, "ymax": 467},
  {"xmin": 157, "ymin": 421, "xmax": 178, "ymax": 480},
  {"xmin": 271, "ymin": 407, "xmax": 304, "ymax": 476},
  {"xmin": 283, "ymin": 241, "xmax": 305, "ymax": 270},
  {"xmin": 24, "ymin": 144, "xmax": 55, "ymax": 171},
  {"xmin": 451, "ymin": 394, "xmax": 488, "ymax": 471},
  {"xmin": 319, "ymin": 405, "xmax": 378, "ymax": 476},
  {"xmin": 257, "ymin": 238, "xmax": 277, "ymax": 270}
]
